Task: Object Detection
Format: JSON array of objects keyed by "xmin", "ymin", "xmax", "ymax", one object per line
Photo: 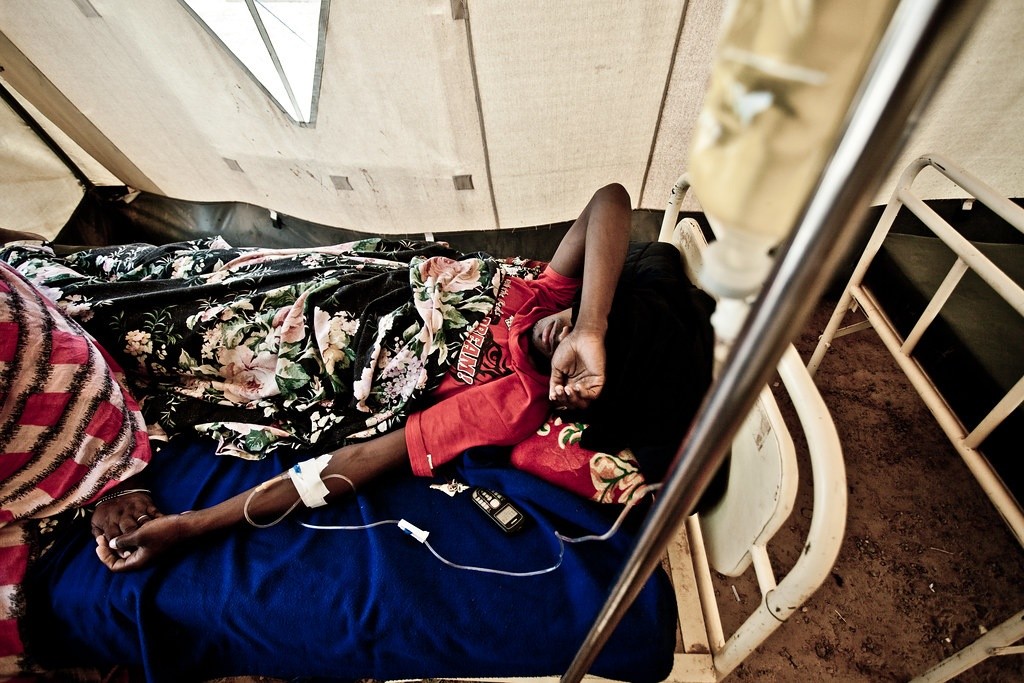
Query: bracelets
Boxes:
[{"xmin": 92, "ymin": 488, "xmax": 152, "ymax": 507}]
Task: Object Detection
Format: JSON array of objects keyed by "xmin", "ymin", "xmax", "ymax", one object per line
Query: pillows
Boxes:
[{"xmin": 572, "ymin": 241, "xmax": 731, "ymax": 520}]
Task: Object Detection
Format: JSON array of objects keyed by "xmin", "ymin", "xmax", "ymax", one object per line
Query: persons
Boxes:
[
  {"xmin": 3, "ymin": 181, "xmax": 715, "ymax": 570},
  {"xmin": 0, "ymin": 254, "xmax": 163, "ymax": 683}
]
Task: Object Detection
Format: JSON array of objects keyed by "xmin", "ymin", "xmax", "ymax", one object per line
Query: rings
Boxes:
[{"xmin": 137, "ymin": 514, "xmax": 150, "ymax": 522}]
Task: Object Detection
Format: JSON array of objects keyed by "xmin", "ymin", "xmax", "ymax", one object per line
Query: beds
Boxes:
[
  {"xmin": 804, "ymin": 154, "xmax": 1024, "ymax": 683},
  {"xmin": 0, "ymin": 187, "xmax": 846, "ymax": 683}
]
